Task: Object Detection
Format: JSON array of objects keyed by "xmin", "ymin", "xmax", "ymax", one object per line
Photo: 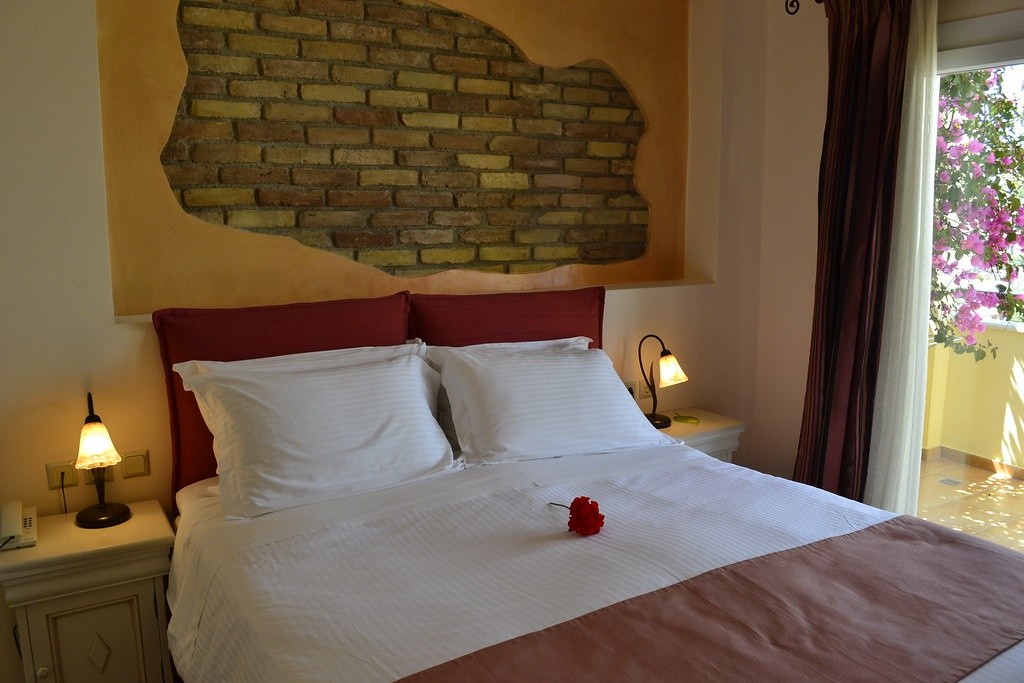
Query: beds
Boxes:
[{"xmin": 152, "ymin": 287, "xmax": 1024, "ymax": 683}]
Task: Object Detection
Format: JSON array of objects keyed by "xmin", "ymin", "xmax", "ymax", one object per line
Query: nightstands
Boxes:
[
  {"xmin": 0, "ymin": 500, "xmax": 175, "ymax": 683},
  {"xmin": 654, "ymin": 407, "xmax": 746, "ymax": 464}
]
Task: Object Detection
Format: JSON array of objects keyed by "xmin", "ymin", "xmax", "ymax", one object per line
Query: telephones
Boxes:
[{"xmin": 0, "ymin": 501, "xmax": 38, "ymax": 552}]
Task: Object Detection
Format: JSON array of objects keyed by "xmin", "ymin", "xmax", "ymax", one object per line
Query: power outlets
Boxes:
[{"xmin": 625, "ymin": 381, "xmax": 637, "ymax": 401}]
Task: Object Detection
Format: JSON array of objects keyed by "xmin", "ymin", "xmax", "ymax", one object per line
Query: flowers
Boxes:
[{"xmin": 549, "ymin": 496, "xmax": 604, "ymax": 536}]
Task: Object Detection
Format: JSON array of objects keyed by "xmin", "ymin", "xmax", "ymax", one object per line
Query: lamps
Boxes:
[
  {"xmin": 638, "ymin": 333, "xmax": 688, "ymax": 429},
  {"xmin": 74, "ymin": 392, "xmax": 130, "ymax": 528}
]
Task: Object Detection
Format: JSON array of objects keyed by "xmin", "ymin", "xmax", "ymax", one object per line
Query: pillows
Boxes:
[{"xmin": 171, "ymin": 337, "xmax": 683, "ymax": 520}]
